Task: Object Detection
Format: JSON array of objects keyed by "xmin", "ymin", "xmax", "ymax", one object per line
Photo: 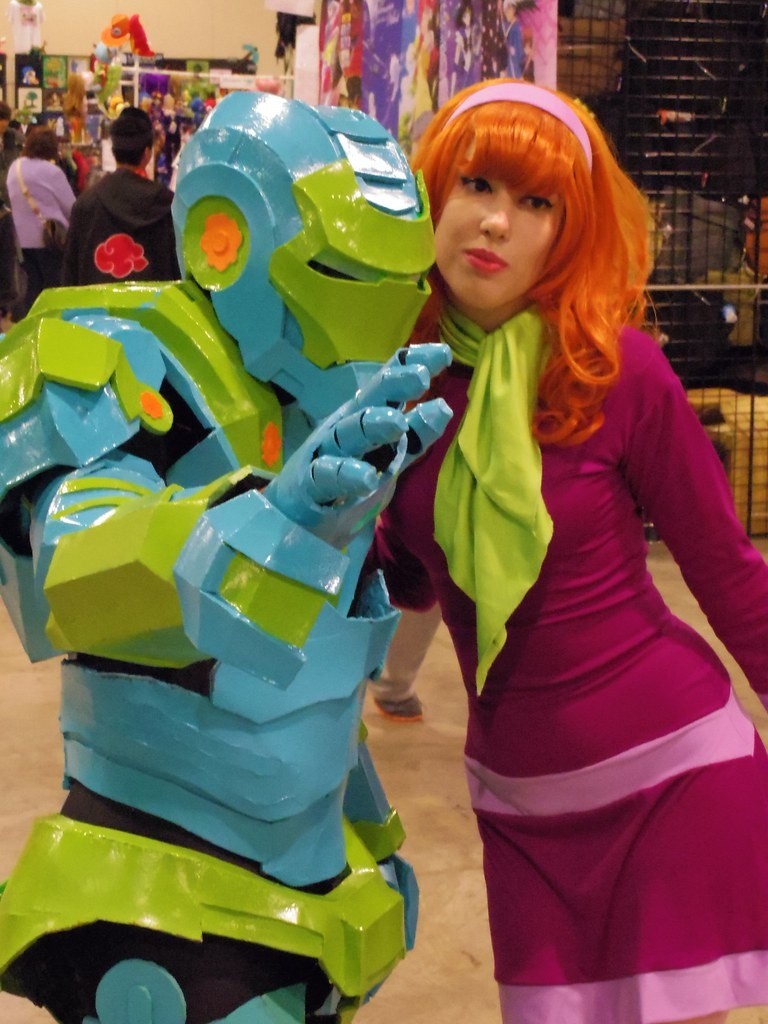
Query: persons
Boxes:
[
  {"xmin": 62, "ymin": 106, "xmax": 181, "ymax": 287},
  {"xmin": 0, "ymin": 92, "xmax": 454, "ymax": 1024},
  {"xmin": 372, "ymin": 596, "xmax": 444, "ymax": 720},
  {"xmin": 370, "ymin": 77, "xmax": 768, "ymax": 1023},
  {"xmin": 0, "ymin": 118, "xmax": 78, "ymax": 324}
]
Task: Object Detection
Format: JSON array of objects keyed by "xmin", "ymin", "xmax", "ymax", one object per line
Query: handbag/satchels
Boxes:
[{"xmin": 43, "ymin": 217, "xmax": 66, "ymax": 250}]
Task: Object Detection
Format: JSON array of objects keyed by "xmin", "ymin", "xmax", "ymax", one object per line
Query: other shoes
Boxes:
[{"xmin": 376, "ymin": 694, "xmax": 422, "ymax": 718}]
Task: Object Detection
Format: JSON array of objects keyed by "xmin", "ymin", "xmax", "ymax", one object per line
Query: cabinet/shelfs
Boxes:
[{"xmin": 14, "ymin": 50, "xmax": 259, "ymax": 200}]
